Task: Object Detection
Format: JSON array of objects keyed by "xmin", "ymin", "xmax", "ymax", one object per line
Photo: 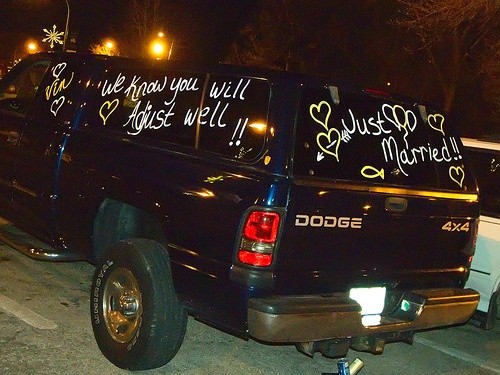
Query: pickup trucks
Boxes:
[{"xmin": 0, "ymin": 49, "xmax": 482, "ymax": 371}]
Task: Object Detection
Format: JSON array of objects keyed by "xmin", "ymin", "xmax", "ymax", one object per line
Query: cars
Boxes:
[{"xmin": 443, "ymin": 135, "xmax": 500, "ymax": 318}]
